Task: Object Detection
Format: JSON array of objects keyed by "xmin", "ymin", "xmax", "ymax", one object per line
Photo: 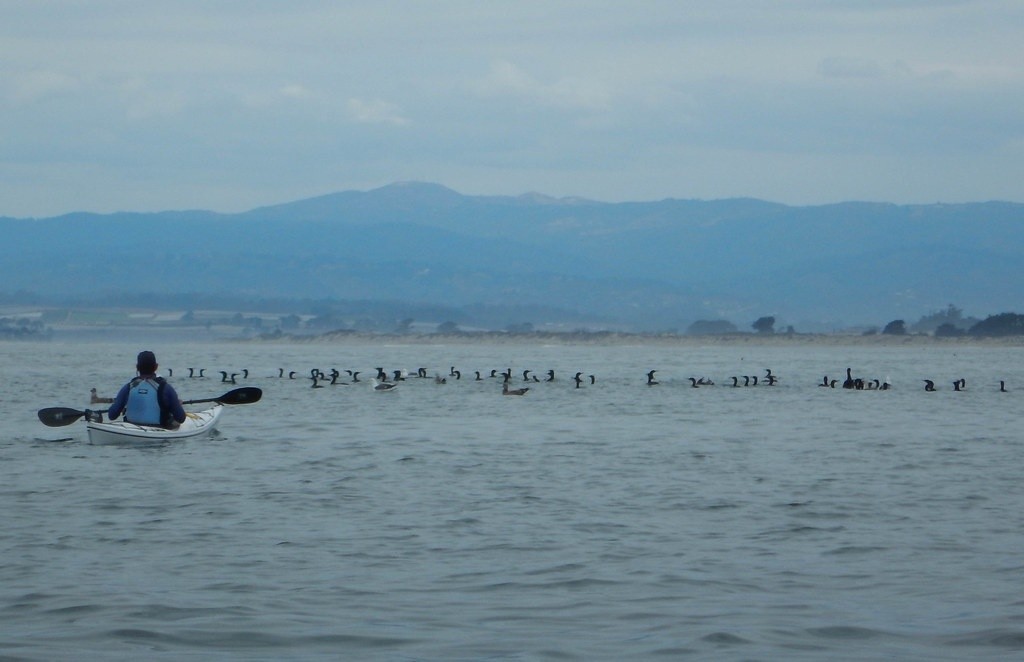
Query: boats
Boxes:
[{"xmin": 86, "ymin": 402, "xmax": 224, "ymax": 449}]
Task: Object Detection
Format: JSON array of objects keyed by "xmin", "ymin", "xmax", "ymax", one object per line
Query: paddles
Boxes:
[{"xmin": 38, "ymin": 386, "xmax": 262, "ymax": 429}]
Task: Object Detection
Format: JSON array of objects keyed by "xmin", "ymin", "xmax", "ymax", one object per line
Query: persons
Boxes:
[{"xmin": 108, "ymin": 351, "xmax": 186, "ymax": 429}]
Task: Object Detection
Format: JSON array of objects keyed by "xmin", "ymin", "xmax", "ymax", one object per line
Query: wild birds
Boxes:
[
  {"xmin": 168, "ymin": 367, "xmax": 1007, "ymax": 395},
  {"xmin": 90, "ymin": 388, "xmax": 113, "ymax": 402}
]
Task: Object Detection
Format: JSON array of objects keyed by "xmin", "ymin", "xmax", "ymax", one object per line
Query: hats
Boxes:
[{"xmin": 137, "ymin": 351, "xmax": 156, "ymax": 374}]
